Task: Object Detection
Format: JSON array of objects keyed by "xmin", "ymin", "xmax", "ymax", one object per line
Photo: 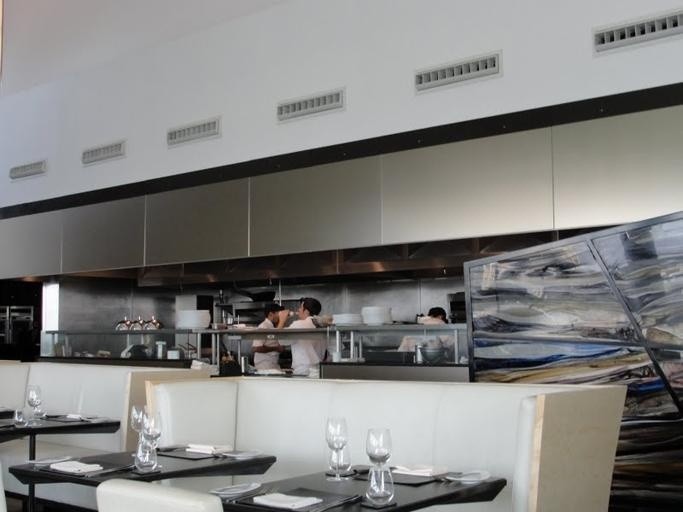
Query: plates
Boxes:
[
  {"xmin": 82, "ymin": 416, "xmax": 110, "ymax": 424},
  {"xmin": 25, "ymin": 456, "xmax": 71, "ymax": 470},
  {"xmin": 175, "ymin": 309, "xmax": 211, "ymax": 330},
  {"xmin": 333, "ymin": 313, "xmax": 363, "ymax": 327},
  {"xmin": 209, "ymin": 482, "xmax": 261, "ymax": 501},
  {"xmin": 360, "ymin": 306, "xmax": 394, "ymax": 327},
  {"xmin": 222, "ymin": 449, "xmax": 262, "ymax": 460},
  {"xmin": 445, "ymin": 470, "xmax": 490, "ymax": 485}
]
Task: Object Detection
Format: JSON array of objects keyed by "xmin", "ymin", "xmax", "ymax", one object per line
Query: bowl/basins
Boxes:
[{"xmin": 419, "ymin": 347, "xmax": 450, "ymax": 364}]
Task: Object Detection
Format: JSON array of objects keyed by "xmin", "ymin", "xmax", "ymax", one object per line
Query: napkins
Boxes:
[
  {"xmin": 66, "ymin": 413, "xmax": 96, "ymax": 419},
  {"xmin": 185, "ymin": 443, "xmax": 233, "ymax": 456},
  {"xmin": 49, "ymin": 459, "xmax": 104, "ymax": 475},
  {"xmin": 253, "ymin": 491, "xmax": 324, "ymax": 510},
  {"xmin": 392, "ymin": 464, "xmax": 448, "ymax": 478}
]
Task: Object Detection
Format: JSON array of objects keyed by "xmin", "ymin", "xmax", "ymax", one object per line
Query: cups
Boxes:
[
  {"xmin": 12, "ymin": 408, "xmax": 29, "ymax": 428},
  {"xmin": 329, "ymin": 446, "xmax": 352, "ymax": 472},
  {"xmin": 366, "ymin": 466, "xmax": 394, "ymax": 505},
  {"xmin": 135, "ymin": 441, "xmax": 157, "ymax": 473},
  {"xmin": 33, "ymin": 400, "xmax": 47, "ymax": 419}
]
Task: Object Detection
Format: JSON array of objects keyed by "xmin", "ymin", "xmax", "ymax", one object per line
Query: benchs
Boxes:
[
  {"xmin": 0, "ymin": 361, "xmax": 211, "ymax": 510},
  {"xmin": 146, "ymin": 377, "xmax": 628, "ymax": 512}
]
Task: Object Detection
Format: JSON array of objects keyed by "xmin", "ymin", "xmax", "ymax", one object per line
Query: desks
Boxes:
[
  {"xmin": 211, "ymin": 462, "xmax": 506, "ymax": 512},
  {"xmin": 0, "ymin": 409, "xmax": 122, "ymax": 509},
  {"xmin": 9, "ymin": 444, "xmax": 276, "ymax": 511}
]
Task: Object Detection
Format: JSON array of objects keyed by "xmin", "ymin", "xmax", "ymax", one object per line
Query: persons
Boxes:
[
  {"xmin": 397, "ymin": 307, "xmax": 455, "ymax": 364},
  {"xmin": 251, "ymin": 304, "xmax": 285, "ymax": 371},
  {"xmin": 277, "ymin": 297, "xmax": 328, "ymax": 369}
]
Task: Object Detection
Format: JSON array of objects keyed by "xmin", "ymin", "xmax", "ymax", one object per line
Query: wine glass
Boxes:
[
  {"xmin": 366, "ymin": 427, "xmax": 392, "ymax": 498},
  {"xmin": 25, "ymin": 384, "xmax": 43, "ymax": 421},
  {"xmin": 130, "ymin": 404, "xmax": 163, "ymax": 465},
  {"xmin": 325, "ymin": 417, "xmax": 350, "ymax": 483}
]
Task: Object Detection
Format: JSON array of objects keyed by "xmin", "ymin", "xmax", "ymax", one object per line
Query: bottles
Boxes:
[
  {"xmin": 286, "ymin": 311, "xmax": 299, "ymax": 319},
  {"xmin": 240, "ymin": 356, "xmax": 249, "ymax": 375}
]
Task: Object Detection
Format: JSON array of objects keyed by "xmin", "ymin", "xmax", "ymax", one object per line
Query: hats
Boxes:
[
  {"xmin": 299, "ymin": 297, "xmax": 321, "ymax": 316},
  {"xmin": 264, "ymin": 303, "xmax": 285, "ymax": 311},
  {"xmin": 428, "ymin": 307, "xmax": 448, "ymax": 324}
]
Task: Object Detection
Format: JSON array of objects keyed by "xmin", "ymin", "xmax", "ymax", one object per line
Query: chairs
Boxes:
[{"xmin": 95, "ymin": 477, "xmax": 222, "ymax": 512}]
